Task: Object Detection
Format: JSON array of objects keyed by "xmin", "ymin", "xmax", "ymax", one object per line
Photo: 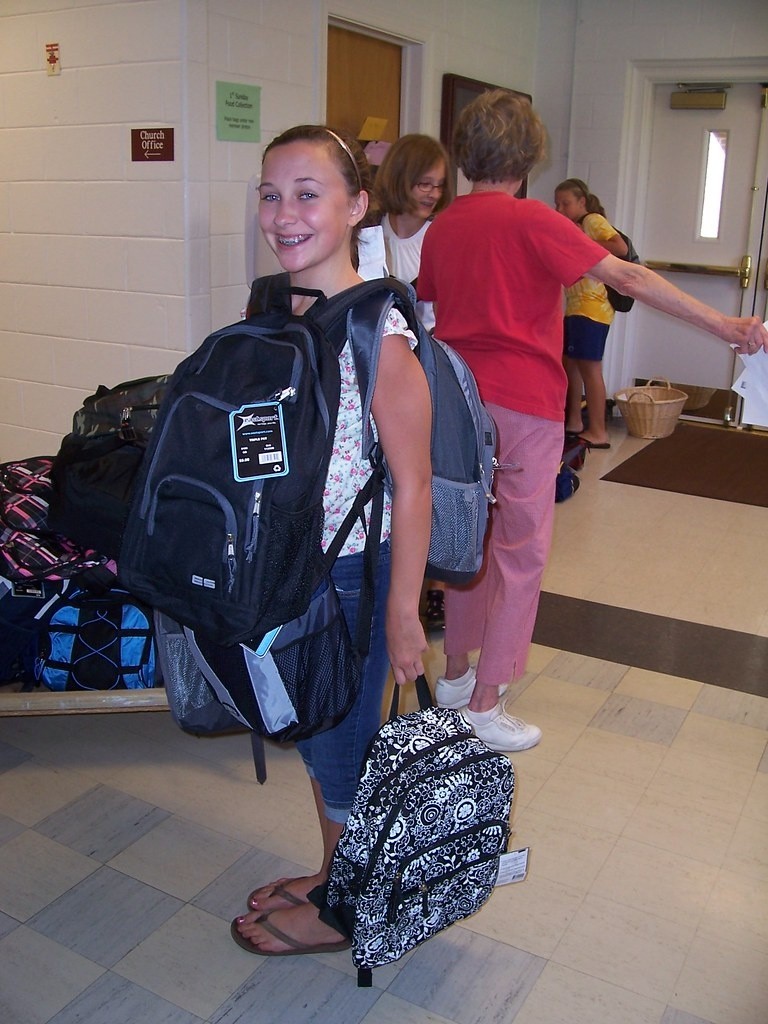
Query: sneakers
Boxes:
[
  {"xmin": 462, "ymin": 698, "xmax": 542, "ymax": 752},
  {"xmin": 425, "ymin": 590, "xmax": 446, "ymax": 632},
  {"xmin": 435, "ymin": 664, "xmax": 509, "ymax": 709}
]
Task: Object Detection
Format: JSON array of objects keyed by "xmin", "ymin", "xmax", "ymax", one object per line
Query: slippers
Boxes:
[
  {"xmin": 247, "ymin": 876, "xmax": 308, "ymax": 912},
  {"xmin": 231, "ymin": 908, "xmax": 353, "ymax": 956},
  {"xmin": 565, "ymin": 431, "xmax": 610, "ymax": 448}
]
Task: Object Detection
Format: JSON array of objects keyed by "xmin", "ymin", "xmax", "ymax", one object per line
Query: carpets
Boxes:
[{"xmin": 600, "ymin": 423, "xmax": 768, "ymax": 508}]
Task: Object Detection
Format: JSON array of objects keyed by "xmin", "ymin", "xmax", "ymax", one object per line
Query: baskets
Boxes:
[{"xmin": 613, "ymin": 377, "xmax": 688, "ymax": 440}]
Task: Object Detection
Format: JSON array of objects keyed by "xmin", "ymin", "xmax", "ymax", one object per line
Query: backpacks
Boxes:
[
  {"xmin": 346, "ymin": 277, "xmax": 519, "ymax": 584},
  {"xmin": 306, "ymin": 673, "xmax": 517, "ymax": 988},
  {"xmin": 154, "ymin": 608, "xmax": 266, "ymax": 785},
  {"xmin": 119, "ymin": 271, "xmax": 421, "ymax": 648},
  {"xmin": 178, "ymin": 461, "xmax": 387, "ymax": 744}
]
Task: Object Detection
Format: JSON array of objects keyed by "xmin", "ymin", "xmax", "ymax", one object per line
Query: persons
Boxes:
[
  {"xmin": 231, "ymin": 126, "xmax": 433, "ymax": 956},
  {"xmin": 350, "ymin": 135, "xmax": 452, "ymax": 334},
  {"xmin": 553, "ymin": 177, "xmax": 628, "ymax": 449},
  {"xmin": 416, "ymin": 87, "xmax": 768, "ymax": 754}
]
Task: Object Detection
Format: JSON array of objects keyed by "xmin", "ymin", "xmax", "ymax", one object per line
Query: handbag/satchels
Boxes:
[
  {"xmin": 561, "ymin": 436, "xmax": 590, "ymax": 471},
  {"xmin": 0, "ymin": 374, "xmax": 173, "ymax": 692},
  {"xmin": 575, "ymin": 212, "xmax": 640, "ymax": 312},
  {"xmin": 554, "ymin": 462, "xmax": 582, "ymax": 504}
]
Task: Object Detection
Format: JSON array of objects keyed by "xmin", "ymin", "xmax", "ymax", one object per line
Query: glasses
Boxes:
[{"xmin": 415, "ymin": 183, "xmax": 445, "ymax": 192}]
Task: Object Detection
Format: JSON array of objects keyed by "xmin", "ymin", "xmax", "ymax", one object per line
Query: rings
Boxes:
[{"xmin": 748, "ymin": 340, "xmax": 756, "ymax": 349}]
